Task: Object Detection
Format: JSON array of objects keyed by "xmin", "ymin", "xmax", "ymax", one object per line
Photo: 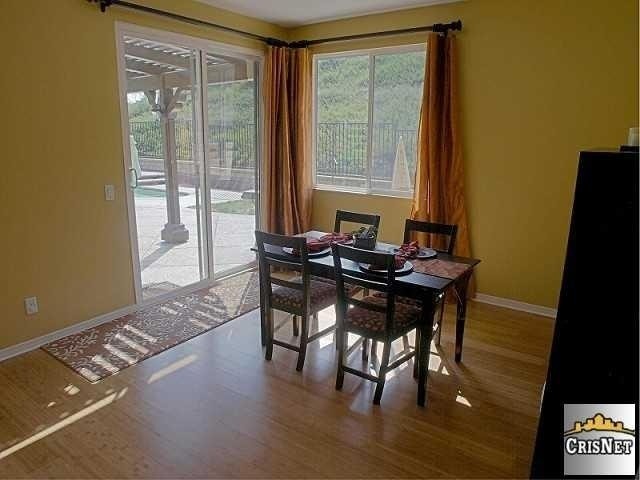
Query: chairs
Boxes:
[{"xmin": 250, "ymin": 209, "xmax": 482, "ymax": 408}]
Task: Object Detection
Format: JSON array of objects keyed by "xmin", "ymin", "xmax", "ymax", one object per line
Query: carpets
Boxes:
[{"xmin": 40, "ymin": 271, "xmax": 282, "ymax": 382}]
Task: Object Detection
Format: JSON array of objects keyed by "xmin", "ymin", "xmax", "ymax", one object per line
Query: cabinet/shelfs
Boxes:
[{"xmin": 529, "ymin": 145, "xmax": 639, "ymax": 478}]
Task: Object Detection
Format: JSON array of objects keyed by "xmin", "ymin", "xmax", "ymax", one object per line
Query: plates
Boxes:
[
  {"xmin": 282, "ymin": 234, "xmax": 354, "ymax": 256},
  {"xmin": 358, "ymin": 246, "xmax": 437, "ymax": 274}
]
